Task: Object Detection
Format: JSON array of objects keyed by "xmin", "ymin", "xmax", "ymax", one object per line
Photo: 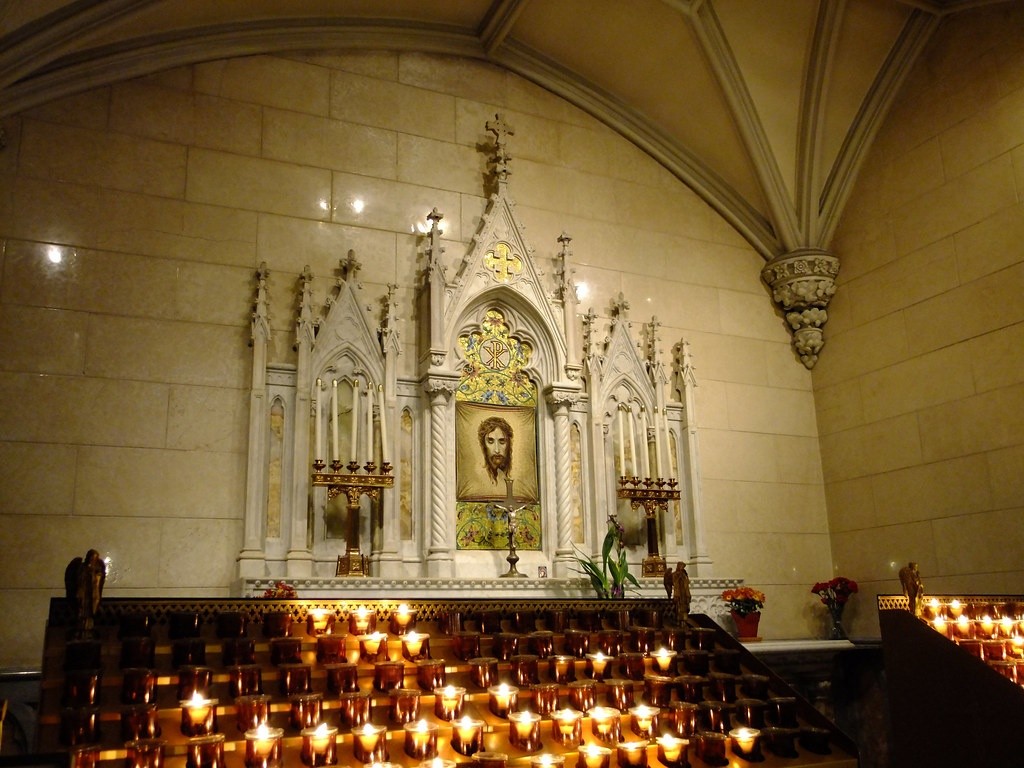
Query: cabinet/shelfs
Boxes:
[
  {"xmin": 36, "ymin": 594, "xmax": 861, "ymax": 767},
  {"xmin": 876, "ymin": 593, "xmax": 1024, "ymax": 768}
]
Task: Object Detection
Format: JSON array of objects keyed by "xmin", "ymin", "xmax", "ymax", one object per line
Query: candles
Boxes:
[
  {"xmin": 180, "ymin": 598, "xmax": 1024, "ymax": 768},
  {"xmin": 316, "ymin": 378, "xmax": 388, "ymax": 463},
  {"xmin": 618, "ymin": 403, "xmax": 674, "ymax": 478}
]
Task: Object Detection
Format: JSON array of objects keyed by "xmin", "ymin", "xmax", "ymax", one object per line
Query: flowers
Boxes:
[
  {"xmin": 720, "ymin": 587, "xmax": 766, "ymax": 619},
  {"xmin": 551, "ymin": 515, "xmax": 642, "ymax": 598},
  {"xmin": 811, "ymin": 577, "xmax": 859, "ymax": 638}
]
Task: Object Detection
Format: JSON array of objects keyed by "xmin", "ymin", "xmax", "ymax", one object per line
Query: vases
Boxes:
[
  {"xmin": 734, "ymin": 613, "xmax": 761, "ymax": 637},
  {"xmin": 829, "ymin": 616, "xmax": 850, "ymax": 640}
]
